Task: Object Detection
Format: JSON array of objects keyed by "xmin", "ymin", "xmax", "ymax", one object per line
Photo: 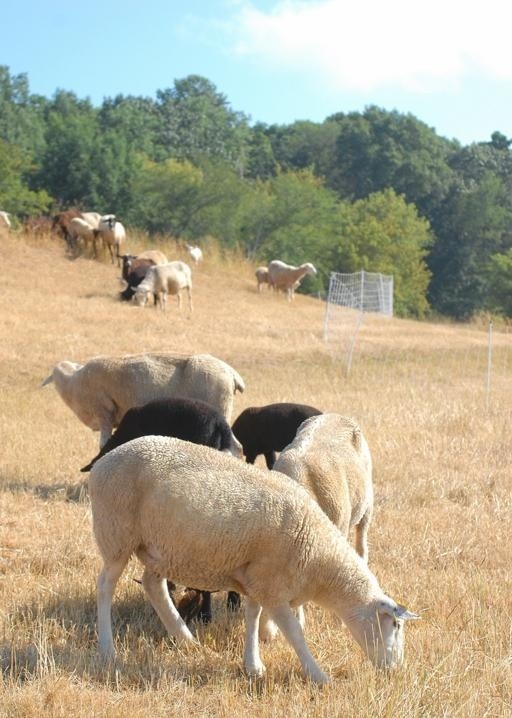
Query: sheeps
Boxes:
[
  {"xmin": 254, "ymin": 260, "xmax": 317, "ymax": 303},
  {"xmin": 38, "ymin": 351, "xmax": 424, "ymax": 691},
  {"xmin": 116, "ymin": 249, "xmax": 194, "ymax": 314},
  {"xmin": 50, "ymin": 211, "xmax": 126, "ymax": 268},
  {"xmin": 186, "ymin": 247, "xmax": 204, "ymax": 265},
  {"xmin": 0, "ymin": 211, "xmax": 11, "ymax": 228}
]
